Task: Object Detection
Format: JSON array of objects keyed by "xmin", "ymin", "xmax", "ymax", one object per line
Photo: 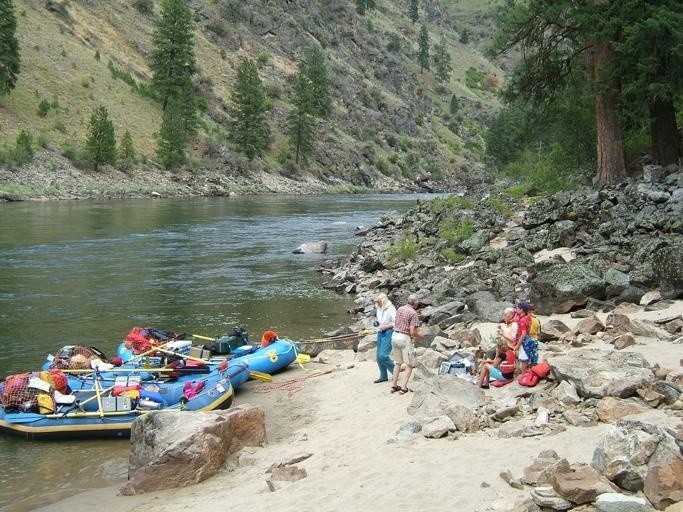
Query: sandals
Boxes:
[{"xmin": 391, "ymin": 386, "xmax": 408, "ymax": 394}]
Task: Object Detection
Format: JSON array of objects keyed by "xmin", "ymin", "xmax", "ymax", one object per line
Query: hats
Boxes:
[{"xmin": 518, "ymin": 301, "xmax": 530, "ymax": 310}]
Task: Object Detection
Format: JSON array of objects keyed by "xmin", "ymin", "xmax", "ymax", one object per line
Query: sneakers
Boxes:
[
  {"xmin": 481, "ymin": 384, "xmax": 489, "ymax": 389},
  {"xmin": 374, "ymin": 378, "xmax": 388, "ymax": 383}
]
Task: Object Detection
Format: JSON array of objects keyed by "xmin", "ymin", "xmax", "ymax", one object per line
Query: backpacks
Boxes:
[{"xmin": 518, "ymin": 362, "xmax": 550, "ymax": 387}]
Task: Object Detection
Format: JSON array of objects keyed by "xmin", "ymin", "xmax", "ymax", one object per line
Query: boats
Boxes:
[
  {"xmin": 295, "ymin": 332, "xmax": 366, "ymax": 356},
  {"xmin": 0, "ymin": 360, "xmax": 250, "ymax": 436},
  {"xmin": 117, "ymin": 339, "xmax": 297, "ymax": 377}
]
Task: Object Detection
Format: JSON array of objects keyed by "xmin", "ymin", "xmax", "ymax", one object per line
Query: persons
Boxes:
[
  {"xmin": 473, "ymin": 299, "xmax": 542, "ymax": 389},
  {"xmin": 371, "ymin": 291, "xmax": 396, "ymax": 384},
  {"xmin": 388, "ymin": 293, "xmax": 425, "ymax": 395}
]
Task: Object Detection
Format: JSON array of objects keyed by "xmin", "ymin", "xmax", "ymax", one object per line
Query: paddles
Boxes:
[
  {"xmin": 252, "ymin": 341, "xmax": 310, "ymax": 362},
  {"xmin": 5, "ymin": 410, "xmax": 136, "ymax": 424},
  {"xmin": 151, "ymin": 344, "xmax": 272, "ymax": 381},
  {"xmin": 91, "ymin": 366, "xmax": 104, "ymax": 419}
]
